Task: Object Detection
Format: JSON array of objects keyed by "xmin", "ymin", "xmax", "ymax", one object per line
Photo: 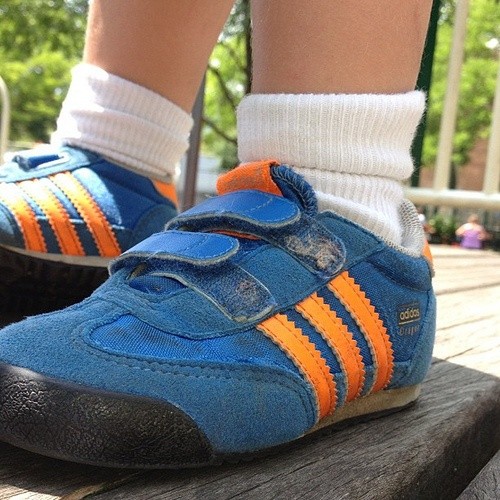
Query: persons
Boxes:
[
  {"xmin": 455, "ymin": 215, "xmax": 492, "ymax": 248},
  {"xmin": 0, "ymin": 0, "xmax": 436, "ymax": 468}
]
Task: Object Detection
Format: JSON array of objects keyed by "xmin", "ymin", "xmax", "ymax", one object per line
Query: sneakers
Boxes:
[
  {"xmin": 0, "ymin": 144, "xmax": 180, "ymax": 314},
  {"xmin": 0, "ymin": 160, "xmax": 436, "ymax": 469}
]
